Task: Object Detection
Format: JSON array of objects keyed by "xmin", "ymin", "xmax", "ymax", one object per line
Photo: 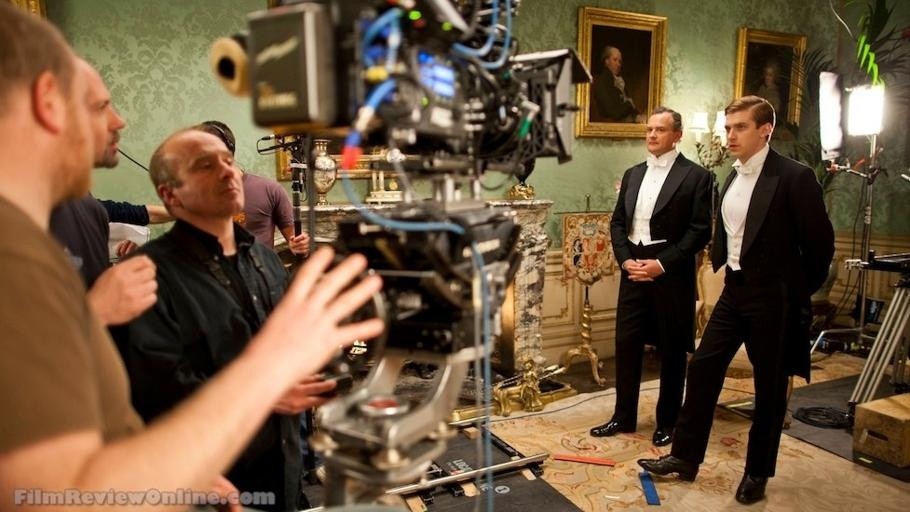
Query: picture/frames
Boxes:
[
  {"xmin": 731, "ymin": 25, "xmax": 808, "ymax": 140},
  {"xmin": 266, "ymin": 0, "xmax": 415, "ymax": 184},
  {"xmin": 574, "ymin": 6, "xmax": 669, "ymax": 139}
]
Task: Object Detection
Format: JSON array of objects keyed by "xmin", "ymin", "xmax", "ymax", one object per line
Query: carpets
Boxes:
[{"xmin": 715, "ymin": 369, "xmax": 910, "ymax": 486}]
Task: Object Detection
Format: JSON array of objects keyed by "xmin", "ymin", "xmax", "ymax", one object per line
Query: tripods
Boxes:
[{"xmin": 812, "ymin": 166, "xmax": 904, "ymax": 364}]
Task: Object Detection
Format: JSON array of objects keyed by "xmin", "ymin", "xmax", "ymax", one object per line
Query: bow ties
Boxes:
[
  {"xmin": 647, "ymin": 156, "xmax": 667, "ymax": 168},
  {"xmin": 735, "ymin": 165, "xmax": 756, "ymax": 176}
]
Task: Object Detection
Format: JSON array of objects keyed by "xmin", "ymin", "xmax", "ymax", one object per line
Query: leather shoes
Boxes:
[
  {"xmin": 637, "ymin": 453, "xmax": 699, "ymax": 482},
  {"xmin": 590, "ymin": 418, "xmax": 636, "ymax": 436},
  {"xmin": 652, "ymin": 428, "xmax": 673, "ymax": 447},
  {"xmin": 735, "ymin": 472, "xmax": 768, "ymax": 504}
]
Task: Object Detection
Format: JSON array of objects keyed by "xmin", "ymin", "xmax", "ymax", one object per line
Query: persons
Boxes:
[
  {"xmin": 591, "ymin": 106, "xmax": 712, "ymax": 448},
  {"xmin": 202, "ymin": 120, "xmax": 313, "ymax": 271},
  {"xmin": 1, "ymin": 2, "xmax": 385, "ymax": 512},
  {"xmin": 589, "ymin": 46, "xmax": 639, "ymax": 123},
  {"xmin": 751, "ymin": 65, "xmax": 780, "ymax": 114},
  {"xmin": 638, "ymin": 94, "xmax": 835, "ymax": 506},
  {"xmin": 107, "ymin": 125, "xmax": 341, "ymax": 512},
  {"xmin": 48, "ymin": 58, "xmax": 176, "ymax": 290}
]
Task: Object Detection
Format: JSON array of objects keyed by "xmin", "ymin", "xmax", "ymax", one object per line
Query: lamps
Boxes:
[{"xmin": 811, "ymin": 84, "xmax": 889, "ymax": 357}]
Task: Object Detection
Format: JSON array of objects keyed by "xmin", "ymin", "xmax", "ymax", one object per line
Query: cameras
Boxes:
[{"xmin": 206, "ymin": 0, "xmax": 594, "ymax": 174}]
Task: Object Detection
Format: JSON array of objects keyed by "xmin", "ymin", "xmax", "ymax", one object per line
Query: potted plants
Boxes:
[{"xmin": 770, "ymin": 1, "xmax": 902, "ymax": 306}]
